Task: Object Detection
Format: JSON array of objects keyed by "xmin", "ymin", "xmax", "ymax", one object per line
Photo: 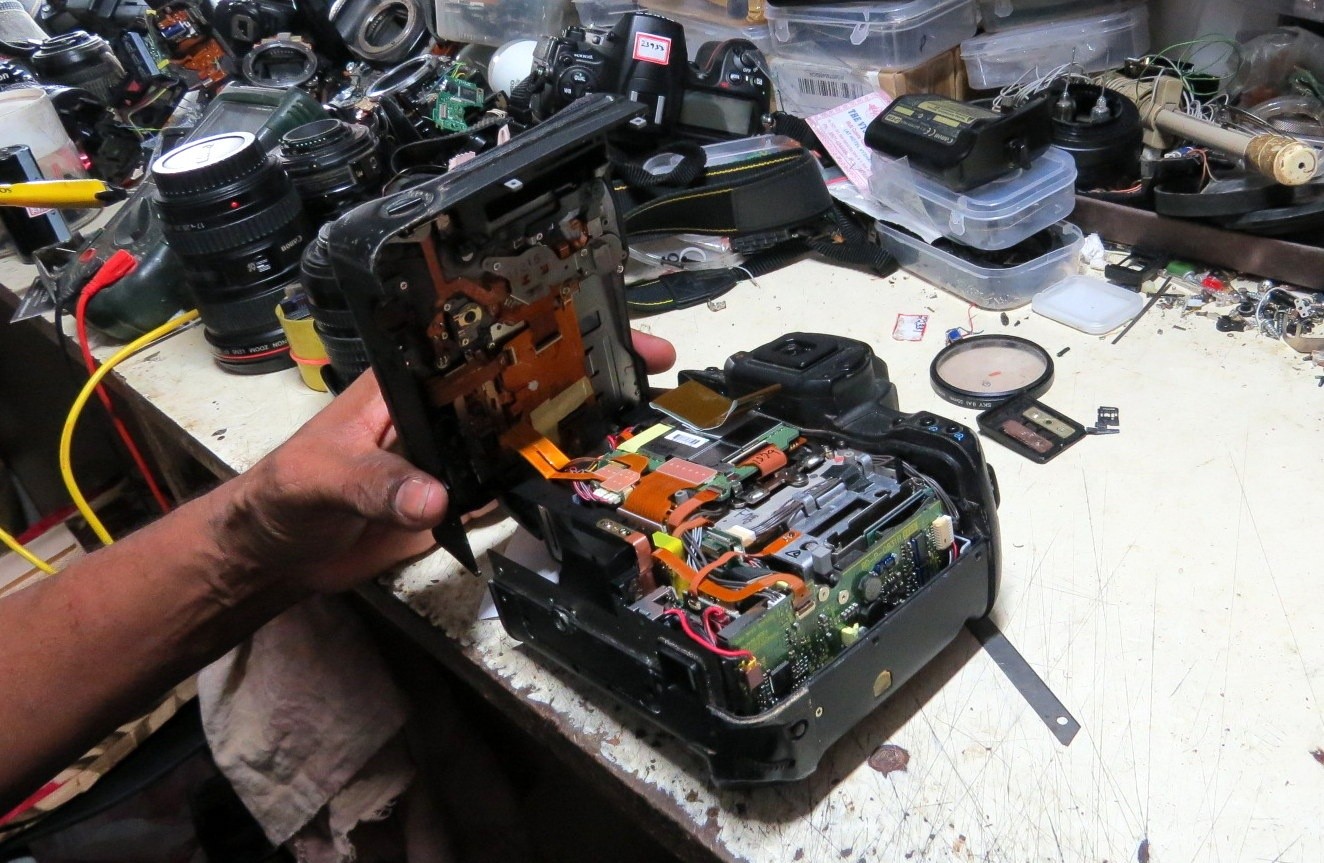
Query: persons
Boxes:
[{"xmin": 0, "ymin": 327, "xmax": 677, "ymax": 820}]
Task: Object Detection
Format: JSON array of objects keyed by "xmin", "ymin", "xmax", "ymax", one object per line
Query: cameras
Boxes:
[{"xmin": 531, "ymin": 9, "xmax": 772, "ymax": 156}]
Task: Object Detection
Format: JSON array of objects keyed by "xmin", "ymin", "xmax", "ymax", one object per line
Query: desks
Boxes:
[{"xmin": 0, "ymin": 217, "xmax": 1324, "ymax": 863}]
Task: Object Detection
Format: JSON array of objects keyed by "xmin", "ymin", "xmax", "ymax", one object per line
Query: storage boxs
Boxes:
[
  {"xmin": 765, "ymin": 0, "xmax": 979, "ymax": 72},
  {"xmin": 871, "ymin": 145, "xmax": 1079, "ymax": 251},
  {"xmin": 771, "ymin": 47, "xmax": 960, "ymax": 119},
  {"xmin": 637, "ymin": 0, "xmax": 770, "ymax": 61},
  {"xmin": 960, "ymin": 9, "xmax": 1151, "ymax": 89},
  {"xmin": 571, "ymin": 0, "xmax": 647, "ymax": 29},
  {"xmin": 872, "ymin": 218, "xmax": 1085, "ymax": 310},
  {"xmin": 979, "ymin": 0, "xmax": 1147, "ymax": 33},
  {"xmin": 433, "ymin": 0, "xmax": 581, "ymax": 49}
]
